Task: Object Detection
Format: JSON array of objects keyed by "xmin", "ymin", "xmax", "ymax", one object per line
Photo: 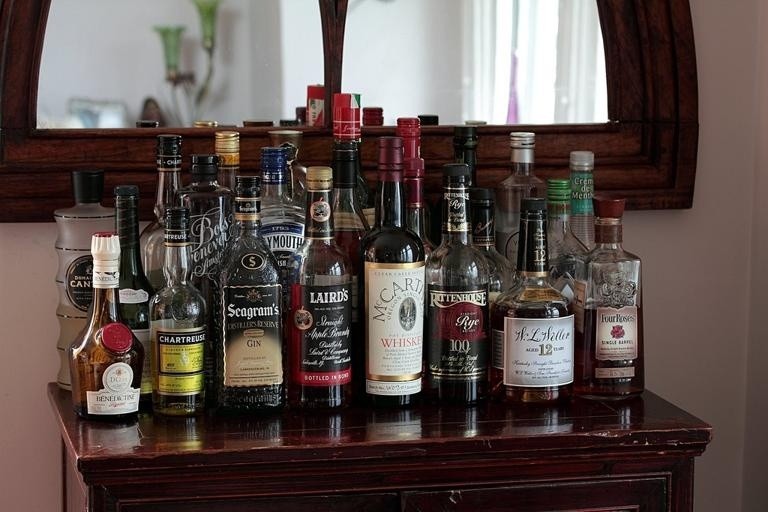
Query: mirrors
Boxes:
[{"xmin": 0, "ymin": 1, "xmax": 701, "ymax": 225}]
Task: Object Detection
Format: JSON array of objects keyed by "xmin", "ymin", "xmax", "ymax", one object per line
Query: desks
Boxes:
[{"xmin": 46, "ymin": 381, "xmax": 713, "ymax": 512}]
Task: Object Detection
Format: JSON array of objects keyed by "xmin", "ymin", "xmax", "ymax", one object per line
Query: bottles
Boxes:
[{"xmin": 49, "ymin": 81, "xmax": 643, "ymax": 426}]
